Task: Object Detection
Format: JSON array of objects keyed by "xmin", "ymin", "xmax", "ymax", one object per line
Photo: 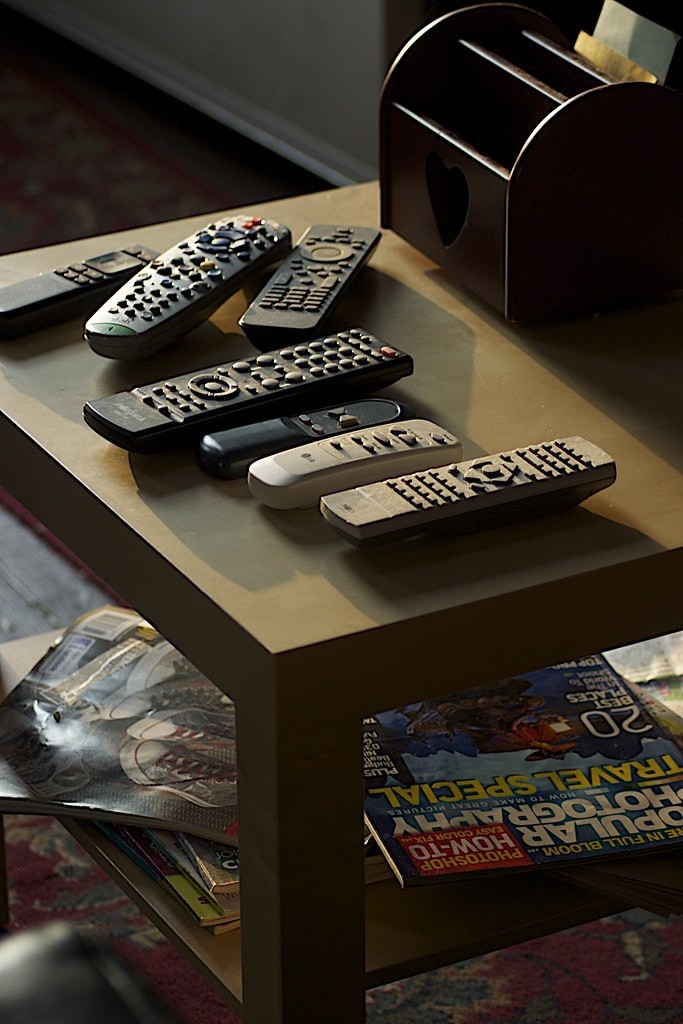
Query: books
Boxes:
[{"xmin": 0, "ymin": 604, "xmax": 683, "ymax": 940}]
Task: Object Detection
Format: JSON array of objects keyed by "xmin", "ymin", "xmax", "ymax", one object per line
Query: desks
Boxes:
[{"xmin": 0, "ymin": 180, "xmax": 683, "ymax": 1024}]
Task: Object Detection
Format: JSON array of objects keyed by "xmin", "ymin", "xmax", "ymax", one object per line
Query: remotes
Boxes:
[{"xmin": 0, "ymin": 213, "xmax": 618, "ymax": 551}]
